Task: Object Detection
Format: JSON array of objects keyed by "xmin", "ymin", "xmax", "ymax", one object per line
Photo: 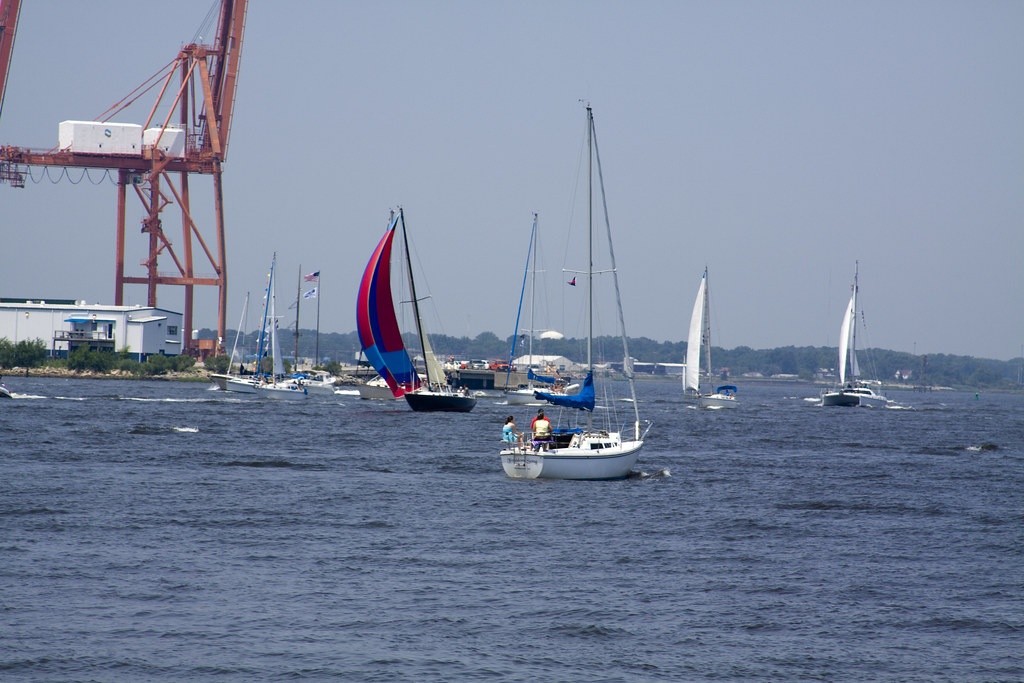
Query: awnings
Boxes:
[{"xmin": 64, "ymin": 319, "xmax": 89, "ymax": 324}]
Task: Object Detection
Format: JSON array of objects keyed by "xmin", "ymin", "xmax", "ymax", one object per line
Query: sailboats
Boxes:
[
  {"xmin": 820, "ymin": 260, "xmax": 883, "ymax": 407},
  {"xmin": 498, "ymin": 96, "xmax": 653, "ymax": 481},
  {"xmin": 681, "ymin": 264, "xmax": 741, "ymax": 409},
  {"xmin": 502, "ymin": 210, "xmax": 580, "ymax": 406},
  {"xmin": 354, "ymin": 205, "xmax": 479, "ymax": 413},
  {"xmin": 205, "ymin": 249, "xmax": 573, "ymax": 401}
]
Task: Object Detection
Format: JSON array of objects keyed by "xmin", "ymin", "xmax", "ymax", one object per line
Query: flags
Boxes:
[
  {"xmin": 303, "ymin": 287, "xmax": 317, "ymax": 299},
  {"xmin": 304, "ymin": 271, "xmax": 319, "ymax": 282}
]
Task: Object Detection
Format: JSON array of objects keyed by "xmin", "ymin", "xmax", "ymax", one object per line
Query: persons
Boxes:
[
  {"xmin": 530, "ymin": 409, "xmax": 553, "ymax": 452},
  {"xmin": 429, "ymin": 383, "xmax": 469, "ymax": 396},
  {"xmin": 502, "ymin": 416, "xmax": 527, "ymax": 450}
]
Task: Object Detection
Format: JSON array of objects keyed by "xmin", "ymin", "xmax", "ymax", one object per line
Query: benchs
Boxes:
[{"xmin": 496, "ymin": 431, "xmax": 558, "ymax": 453}]
